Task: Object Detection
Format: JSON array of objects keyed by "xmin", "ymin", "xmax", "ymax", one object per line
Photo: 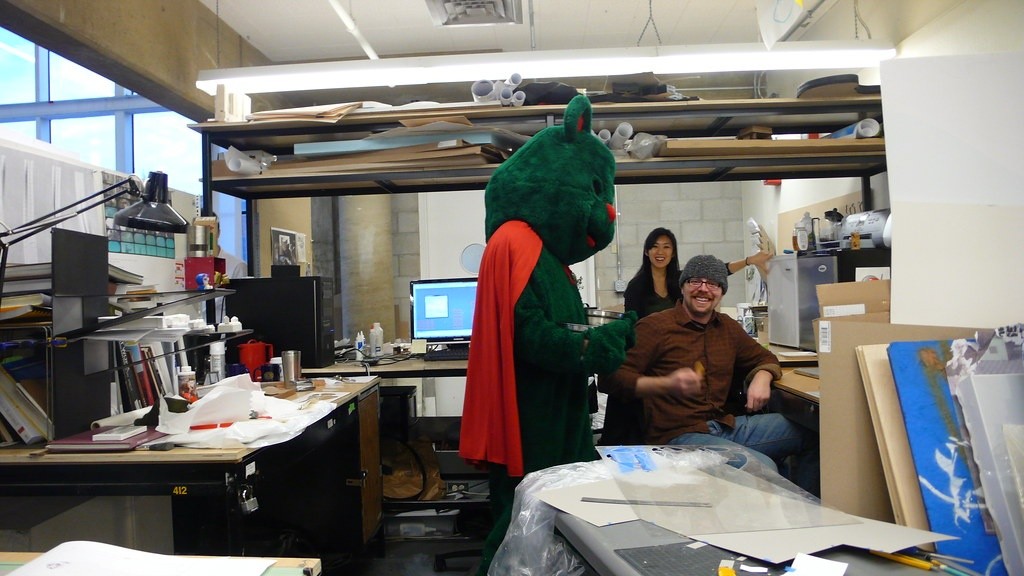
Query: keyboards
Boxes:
[{"xmin": 424, "ymin": 349, "xmax": 469, "ymax": 361}]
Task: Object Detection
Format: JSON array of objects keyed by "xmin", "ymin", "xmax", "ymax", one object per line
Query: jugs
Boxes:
[{"xmin": 238, "ymin": 340, "xmax": 274, "ymax": 379}]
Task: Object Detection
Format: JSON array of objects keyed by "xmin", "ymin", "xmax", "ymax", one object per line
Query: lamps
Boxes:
[
  {"xmin": 194, "ymin": 1, "xmax": 896, "ymax": 95},
  {"xmin": 0, "ymin": 170, "xmax": 190, "ymax": 309}
]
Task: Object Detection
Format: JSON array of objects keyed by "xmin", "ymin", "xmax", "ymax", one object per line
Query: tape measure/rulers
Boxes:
[{"xmin": 581, "ymin": 497, "xmax": 712, "ymax": 507}]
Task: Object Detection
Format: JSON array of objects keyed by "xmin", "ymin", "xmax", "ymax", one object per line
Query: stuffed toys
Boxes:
[{"xmin": 460, "ymin": 95, "xmax": 638, "ymax": 576}]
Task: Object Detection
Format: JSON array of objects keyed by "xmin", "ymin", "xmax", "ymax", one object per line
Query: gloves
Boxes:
[{"xmin": 579, "ymin": 311, "xmax": 638, "ymax": 378}]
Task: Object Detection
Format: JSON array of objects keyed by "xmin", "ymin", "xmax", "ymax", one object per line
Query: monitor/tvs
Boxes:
[{"xmin": 409, "ymin": 277, "xmax": 479, "ymax": 349}]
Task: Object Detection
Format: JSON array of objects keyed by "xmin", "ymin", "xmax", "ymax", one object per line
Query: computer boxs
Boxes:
[{"xmin": 226, "ymin": 276, "xmax": 334, "ymax": 368}]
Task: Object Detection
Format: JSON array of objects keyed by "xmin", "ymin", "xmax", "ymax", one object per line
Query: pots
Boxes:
[{"xmin": 581, "ymin": 303, "xmax": 624, "ymax": 326}]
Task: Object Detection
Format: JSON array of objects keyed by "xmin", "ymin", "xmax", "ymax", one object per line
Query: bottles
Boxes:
[
  {"xmin": 354, "ymin": 330, "xmax": 366, "ymax": 361},
  {"xmin": 209, "ymin": 342, "xmax": 225, "ymax": 382},
  {"xmin": 178, "ymin": 366, "xmax": 198, "ymax": 404},
  {"xmin": 792, "ymin": 208, "xmax": 843, "ymax": 251},
  {"xmin": 369, "ymin": 323, "xmax": 384, "ymax": 357}
]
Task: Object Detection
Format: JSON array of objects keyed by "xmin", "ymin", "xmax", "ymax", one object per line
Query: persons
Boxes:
[
  {"xmin": 623, "ymin": 228, "xmax": 774, "ymax": 317},
  {"xmin": 595, "ymin": 255, "xmax": 820, "ymax": 473}
]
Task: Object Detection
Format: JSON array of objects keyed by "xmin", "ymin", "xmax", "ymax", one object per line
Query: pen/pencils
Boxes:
[
  {"xmin": 929, "ymin": 559, "xmax": 973, "ymax": 576},
  {"xmin": 189, "ymin": 423, "xmax": 233, "ymax": 430}
]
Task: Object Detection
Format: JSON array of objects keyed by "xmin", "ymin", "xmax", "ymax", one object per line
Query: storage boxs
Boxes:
[{"xmin": 430, "ymin": 441, "xmax": 488, "ymax": 474}]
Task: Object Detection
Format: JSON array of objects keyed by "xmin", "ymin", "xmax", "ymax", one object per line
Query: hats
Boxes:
[{"xmin": 679, "ymin": 255, "xmax": 728, "ymax": 295}]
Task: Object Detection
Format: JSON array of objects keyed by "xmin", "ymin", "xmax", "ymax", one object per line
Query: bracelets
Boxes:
[{"xmin": 745, "ymin": 256, "xmax": 749, "ymax": 266}]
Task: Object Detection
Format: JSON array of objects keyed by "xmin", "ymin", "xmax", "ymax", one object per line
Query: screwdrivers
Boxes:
[{"xmin": 899, "ymin": 546, "xmax": 975, "ymax": 568}]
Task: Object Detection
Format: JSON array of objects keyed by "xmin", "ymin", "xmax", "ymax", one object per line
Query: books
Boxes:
[{"xmin": 0, "ymin": 262, "xmax": 191, "ymax": 451}]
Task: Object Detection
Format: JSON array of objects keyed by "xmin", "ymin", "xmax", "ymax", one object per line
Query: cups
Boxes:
[
  {"xmin": 270, "ymin": 356, "xmax": 284, "ymax": 380},
  {"xmin": 253, "ymin": 364, "xmax": 280, "ymax": 381},
  {"xmin": 281, "ymin": 351, "xmax": 301, "ymax": 388}
]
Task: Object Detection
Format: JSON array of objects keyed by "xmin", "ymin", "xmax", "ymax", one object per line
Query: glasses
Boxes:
[{"xmin": 685, "ymin": 279, "xmax": 720, "ymax": 289}]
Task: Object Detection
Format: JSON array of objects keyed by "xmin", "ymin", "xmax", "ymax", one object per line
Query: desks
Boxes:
[
  {"xmin": 0, "ymin": 347, "xmax": 469, "ymax": 576},
  {"xmin": 751, "ymin": 338, "xmax": 819, "ymax": 429}
]
